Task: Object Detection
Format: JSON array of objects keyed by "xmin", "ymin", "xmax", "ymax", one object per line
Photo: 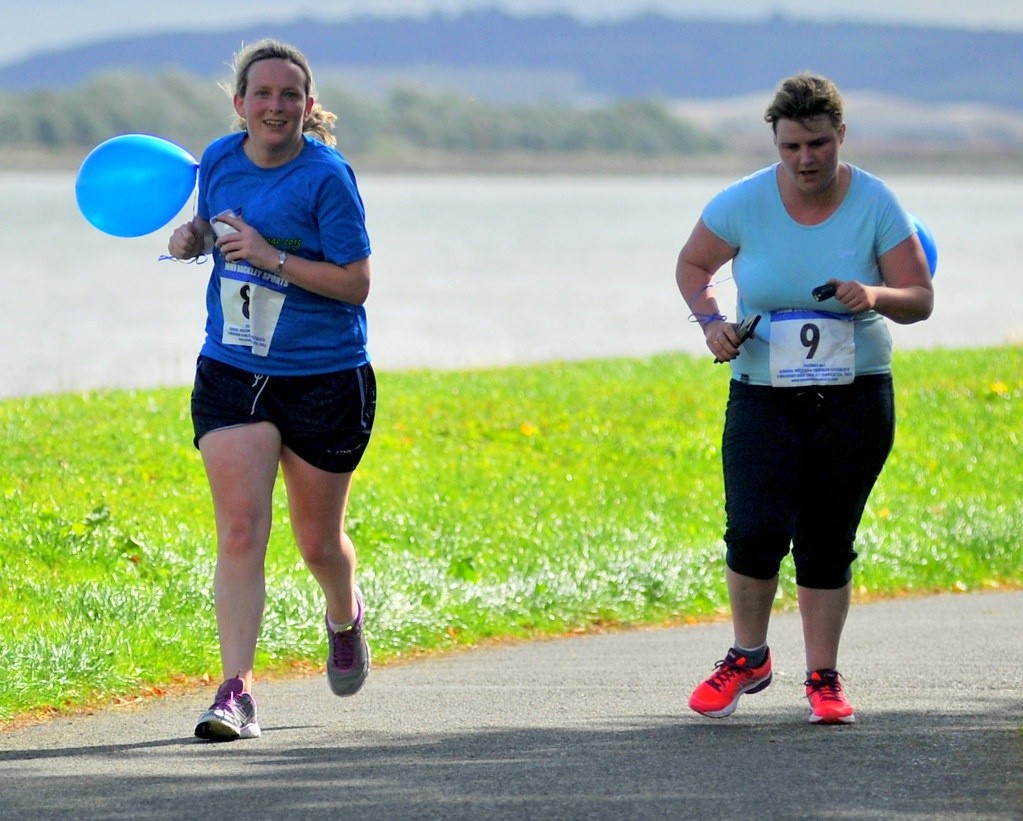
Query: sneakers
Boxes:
[
  {"xmin": 325, "ymin": 583, "xmax": 371, "ymax": 698},
  {"xmin": 802, "ymin": 668, "xmax": 856, "ymax": 724},
  {"xmin": 194, "ymin": 678, "xmax": 262, "ymax": 741},
  {"xmin": 689, "ymin": 645, "xmax": 773, "ymax": 718}
]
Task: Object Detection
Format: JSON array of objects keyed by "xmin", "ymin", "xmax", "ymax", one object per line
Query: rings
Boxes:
[{"xmin": 712, "ymin": 339, "xmax": 719, "ymax": 345}]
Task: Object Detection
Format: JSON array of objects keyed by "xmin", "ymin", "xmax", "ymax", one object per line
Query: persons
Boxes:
[
  {"xmin": 168, "ymin": 37, "xmax": 380, "ymax": 739},
  {"xmin": 676, "ymin": 72, "xmax": 936, "ymax": 722}
]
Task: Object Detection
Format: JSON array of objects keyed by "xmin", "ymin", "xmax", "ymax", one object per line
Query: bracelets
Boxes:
[{"xmin": 274, "ymin": 249, "xmax": 288, "ymax": 277}]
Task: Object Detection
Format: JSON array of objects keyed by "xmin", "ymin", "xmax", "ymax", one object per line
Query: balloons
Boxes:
[
  {"xmin": 897, "ymin": 207, "xmax": 939, "ymax": 283},
  {"xmin": 73, "ymin": 133, "xmax": 201, "ymax": 238}
]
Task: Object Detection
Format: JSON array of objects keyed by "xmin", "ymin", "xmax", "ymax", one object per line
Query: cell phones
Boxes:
[
  {"xmin": 209, "ymin": 209, "xmax": 244, "ymax": 263},
  {"xmin": 715, "ymin": 314, "xmax": 761, "ymax": 363}
]
